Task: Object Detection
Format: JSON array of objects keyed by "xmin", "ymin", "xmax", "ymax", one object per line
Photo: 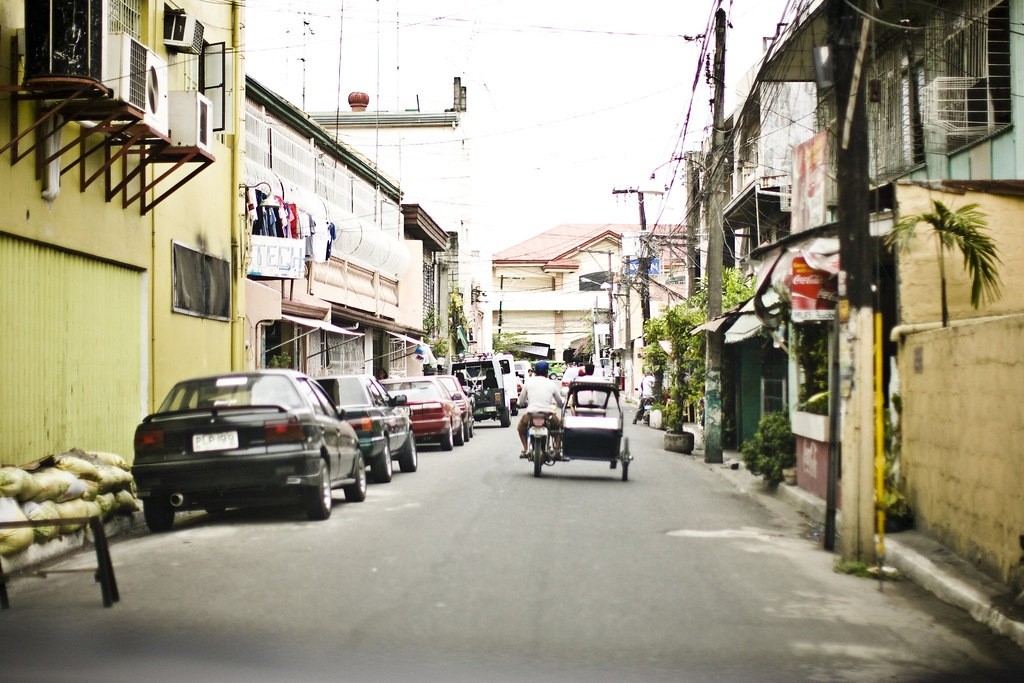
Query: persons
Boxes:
[
  {"xmin": 517, "ymin": 362, "xmax": 564, "ymax": 457},
  {"xmin": 614, "ymin": 362, "xmax": 621, "ymax": 384},
  {"xmin": 575, "ymin": 364, "xmax": 603, "ymax": 405},
  {"xmin": 633, "ymin": 371, "xmax": 655, "ymax": 424}
]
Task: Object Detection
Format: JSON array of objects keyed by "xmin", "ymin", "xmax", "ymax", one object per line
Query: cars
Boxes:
[
  {"xmin": 311, "ymin": 372, "xmax": 418, "ymax": 484},
  {"xmin": 131, "ymin": 367, "xmax": 367, "ymax": 533},
  {"xmin": 378, "ymin": 355, "xmax": 530, "ymax": 453}
]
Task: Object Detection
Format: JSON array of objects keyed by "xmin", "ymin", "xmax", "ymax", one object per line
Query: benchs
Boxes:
[{"xmin": 562, "ymin": 415, "xmax": 618, "ymax": 430}]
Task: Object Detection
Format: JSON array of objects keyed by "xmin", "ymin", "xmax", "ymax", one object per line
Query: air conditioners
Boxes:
[
  {"xmin": 107, "ymin": 31, "xmax": 168, "ymax": 140},
  {"xmin": 167, "ymin": 90, "xmax": 213, "ymax": 154},
  {"xmin": 165, "ymin": 14, "xmax": 205, "ymax": 54}
]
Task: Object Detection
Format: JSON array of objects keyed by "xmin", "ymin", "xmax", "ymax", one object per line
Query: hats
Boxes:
[{"xmin": 535, "ymin": 361, "xmax": 549, "ymax": 372}]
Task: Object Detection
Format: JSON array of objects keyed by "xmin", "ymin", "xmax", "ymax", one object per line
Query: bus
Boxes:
[{"xmin": 531, "ymin": 360, "xmax": 567, "ymax": 380}]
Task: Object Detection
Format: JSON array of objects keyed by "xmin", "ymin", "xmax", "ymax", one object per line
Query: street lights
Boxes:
[{"xmin": 580, "ymin": 277, "xmax": 614, "ymax": 349}]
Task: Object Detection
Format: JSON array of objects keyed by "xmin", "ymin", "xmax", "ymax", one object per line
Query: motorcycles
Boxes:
[
  {"xmin": 529, "ymin": 375, "xmax": 634, "ymax": 482},
  {"xmin": 635, "ymin": 387, "xmax": 654, "ymax": 424}
]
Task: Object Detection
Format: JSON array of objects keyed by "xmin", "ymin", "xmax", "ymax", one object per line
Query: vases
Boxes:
[{"xmin": 783, "ymin": 466, "xmax": 797, "ymax": 485}]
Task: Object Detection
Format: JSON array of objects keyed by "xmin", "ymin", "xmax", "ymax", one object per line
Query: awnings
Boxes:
[
  {"xmin": 260, "ymin": 313, "xmax": 431, "ymax": 361},
  {"xmin": 687, "ymin": 288, "xmax": 782, "ymax": 344}
]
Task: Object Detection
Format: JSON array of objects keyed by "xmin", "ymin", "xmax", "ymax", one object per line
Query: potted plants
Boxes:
[
  {"xmin": 663, "ymin": 306, "xmax": 698, "ymax": 453},
  {"xmin": 649, "ymin": 403, "xmax": 665, "ymax": 429}
]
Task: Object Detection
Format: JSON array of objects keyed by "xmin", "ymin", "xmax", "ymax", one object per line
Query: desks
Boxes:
[{"xmin": 666, "ymin": 398, "xmax": 690, "ymax": 423}]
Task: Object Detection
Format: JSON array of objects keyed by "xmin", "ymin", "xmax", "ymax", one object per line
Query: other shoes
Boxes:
[
  {"xmin": 519, "ymin": 451, "xmax": 530, "ymax": 459},
  {"xmin": 554, "ymin": 453, "xmax": 562, "ymax": 461},
  {"xmin": 632, "ymin": 418, "xmax": 638, "ymax": 424}
]
